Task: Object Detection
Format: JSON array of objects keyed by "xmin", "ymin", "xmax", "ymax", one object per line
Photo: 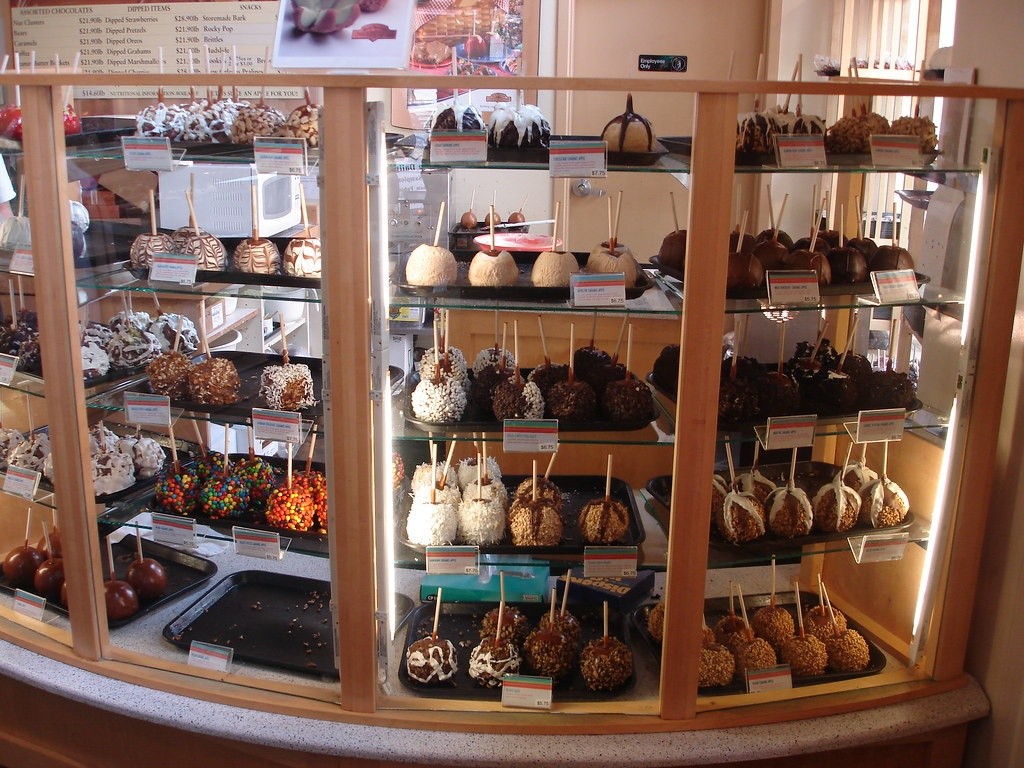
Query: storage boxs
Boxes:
[
  {"xmin": 98, "ymin": 298, "xmax": 227, "ymax": 336},
  {"xmin": 79, "ymin": 186, "xmax": 135, "ymax": 219}
]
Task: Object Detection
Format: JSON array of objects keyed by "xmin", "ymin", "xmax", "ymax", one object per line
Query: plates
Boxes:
[
  {"xmin": 472, "ymin": 233, "xmax": 563, "ymax": 251},
  {"xmin": 453, "ymin": 41, "xmax": 513, "ymax": 63},
  {"xmin": 409, "ymin": 55, "xmax": 452, "ymax": 67},
  {"xmin": 444, "ymin": 64, "xmax": 497, "ymax": 76},
  {"xmin": 499, "ymin": 58, "xmax": 518, "ymax": 76}
]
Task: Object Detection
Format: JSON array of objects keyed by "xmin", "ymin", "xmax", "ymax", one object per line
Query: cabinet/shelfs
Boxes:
[{"xmin": 0, "ymin": 66, "xmax": 1024, "ymax": 736}]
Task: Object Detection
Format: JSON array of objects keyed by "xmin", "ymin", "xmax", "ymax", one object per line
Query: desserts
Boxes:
[{"xmin": 0, "ymin": 95, "xmax": 939, "ymax": 693}]
